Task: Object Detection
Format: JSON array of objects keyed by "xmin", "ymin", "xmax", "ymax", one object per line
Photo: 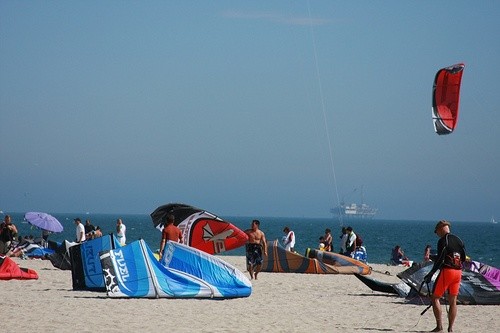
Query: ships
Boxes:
[{"xmin": 330, "ymin": 185, "xmax": 379, "ymax": 216}]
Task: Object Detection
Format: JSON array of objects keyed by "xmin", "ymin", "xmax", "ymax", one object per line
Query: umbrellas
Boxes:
[{"xmin": 24, "ymin": 211, "xmax": 64, "ymax": 234}]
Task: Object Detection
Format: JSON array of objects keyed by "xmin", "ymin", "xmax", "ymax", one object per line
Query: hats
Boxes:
[{"xmin": 434, "ymin": 220, "xmax": 450, "ymax": 233}]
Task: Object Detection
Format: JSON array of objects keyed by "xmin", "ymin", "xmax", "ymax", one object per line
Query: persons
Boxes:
[
  {"xmin": 1, "ymin": 214, "xmax": 18, "ymax": 254},
  {"xmin": 282, "ymin": 226, "xmax": 296, "ymax": 252},
  {"xmin": 243, "ymin": 219, "xmax": 268, "ymax": 280},
  {"xmin": 116, "ymin": 218, "xmax": 126, "ymax": 245},
  {"xmin": 92, "ymin": 226, "xmax": 103, "ymax": 238},
  {"xmin": 43, "ymin": 230, "xmax": 49, "ymax": 250},
  {"xmin": 354, "ymin": 238, "xmax": 367, "ymax": 262},
  {"xmin": 392, "ymin": 246, "xmax": 404, "ymax": 264},
  {"xmin": 74, "ymin": 217, "xmax": 85, "ymax": 243},
  {"xmin": 83, "ymin": 220, "xmax": 94, "ymax": 235},
  {"xmin": 160, "ymin": 214, "xmax": 183, "ymax": 256},
  {"xmin": 319, "ymin": 236, "xmax": 327, "ymax": 248},
  {"xmin": 324, "ymin": 229, "xmax": 333, "ymax": 252},
  {"xmin": 346, "ymin": 227, "xmax": 357, "ymax": 253},
  {"xmin": 340, "ymin": 226, "xmax": 348, "ymax": 254},
  {"xmin": 424, "ymin": 219, "xmax": 466, "ymax": 333},
  {"xmin": 425, "ymin": 245, "xmax": 431, "ymax": 259}
]
